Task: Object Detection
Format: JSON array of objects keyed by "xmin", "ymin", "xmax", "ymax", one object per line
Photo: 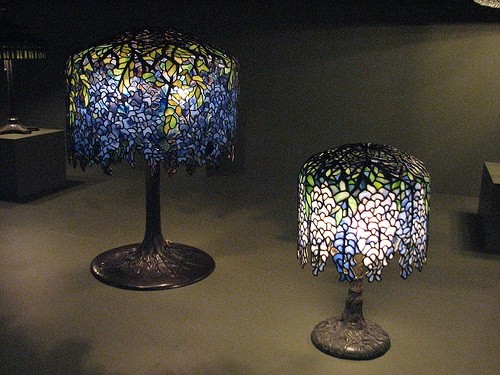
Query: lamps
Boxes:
[
  {"xmin": 0, "ymin": 14, "xmax": 48, "ymax": 135},
  {"xmin": 65, "ymin": 28, "xmax": 242, "ymax": 290},
  {"xmin": 295, "ymin": 142, "xmax": 431, "ymax": 361}
]
